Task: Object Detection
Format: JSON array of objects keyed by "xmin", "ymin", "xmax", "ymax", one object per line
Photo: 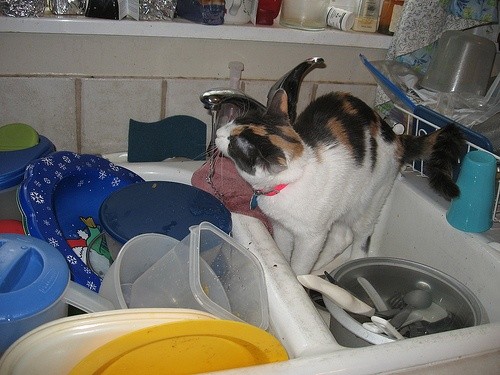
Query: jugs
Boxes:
[{"xmin": 0, "ymin": 233, "xmax": 116, "ymax": 354}]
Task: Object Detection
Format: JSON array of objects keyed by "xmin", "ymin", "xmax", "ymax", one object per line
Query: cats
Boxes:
[{"xmin": 210, "ymin": 87, "xmax": 463, "ymax": 286}]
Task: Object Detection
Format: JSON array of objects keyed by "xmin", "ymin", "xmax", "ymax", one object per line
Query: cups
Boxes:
[
  {"xmin": 417, "ymin": 31, "xmax": 499, "ymax": 96},
  {"xmin": 131, "ymin": 221, "xmax": 270, "ymax": 331},
  {"xmin": 447, "ymin": 150, "xmax": 496, "ymax": 232},
  {"xmin": 224, "ymin": 0, "xmax": 329, "ymax": 31}
]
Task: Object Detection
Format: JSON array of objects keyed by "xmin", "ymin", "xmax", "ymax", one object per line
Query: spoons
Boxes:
[{"xmin": 389, "ymin": 290, "xmax": 433, "ymax": 328}]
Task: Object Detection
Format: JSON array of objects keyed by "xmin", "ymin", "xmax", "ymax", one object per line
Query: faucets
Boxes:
[{"xmin": 200, "ymin": 57, "xmax": 324, "ymax": 124}]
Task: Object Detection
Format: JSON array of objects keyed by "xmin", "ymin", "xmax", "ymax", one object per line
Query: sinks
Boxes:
[
  {"xmin": 0, "ymin": 152, "xmax": 318, "ymax": 375},
  {"xmin": 236, "ymin": 162, "xmax": 500, "ymax": 356}
]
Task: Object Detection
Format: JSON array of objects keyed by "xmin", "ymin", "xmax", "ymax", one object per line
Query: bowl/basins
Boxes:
[
  {"xmin": 87, "ymin": 181, "xmax": 232, "ymax": 278},
  {"xmin": 99, "ymin": 233, "xmax": 232, "ymax": 313}
]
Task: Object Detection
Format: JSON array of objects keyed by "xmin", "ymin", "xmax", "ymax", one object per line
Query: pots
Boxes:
[{"xmin": 323, "ymin": 257, "xmax": 488, "ymax": 348}]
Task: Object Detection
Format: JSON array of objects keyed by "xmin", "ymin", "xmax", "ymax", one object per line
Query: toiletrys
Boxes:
[
  {"xmin": 357, "ymin": 0, "xmax": 381, "ymax": 29},
  {"xmin": 229, "ymin": 62, "xmax": 243, "ymax": 89}
]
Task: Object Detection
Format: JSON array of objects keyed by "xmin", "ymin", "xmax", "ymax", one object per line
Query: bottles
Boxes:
[
  {"xmin": 0, "ymin": 123, "xmax": 57, "ymax": 222},
  {"xmin": 326, "ymin": 0, "xmax": 403, "ymax": 36}
]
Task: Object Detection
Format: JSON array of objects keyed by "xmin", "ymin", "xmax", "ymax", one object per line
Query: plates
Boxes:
[
  {"xmin": 67, "ymin": 319, "xmax": 288, "ymax": 374},
  {"xmin": 15, "ymin": 151, "xmax": 146, "ymax": 293}
]
